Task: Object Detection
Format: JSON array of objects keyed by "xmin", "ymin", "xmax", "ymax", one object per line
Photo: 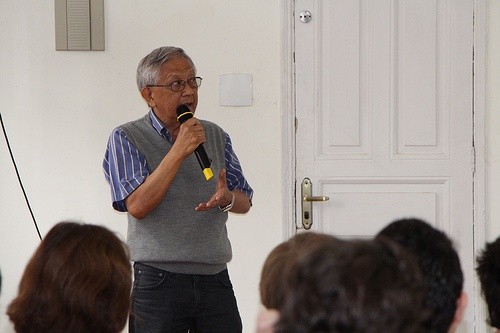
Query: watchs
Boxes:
[{"xmin": 219, "ymin": 192, "xmax": 235, "ymax": 212}]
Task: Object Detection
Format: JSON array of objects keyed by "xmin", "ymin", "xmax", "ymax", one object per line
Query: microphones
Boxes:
[{"xmin": 176, "ymin": 104, "xmax": 213, "ymax": 181}]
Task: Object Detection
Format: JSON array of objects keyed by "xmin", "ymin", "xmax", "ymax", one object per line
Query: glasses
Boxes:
[{"xmin": 146, "ymin": 77, "xmax": 203, "ymax": 92}]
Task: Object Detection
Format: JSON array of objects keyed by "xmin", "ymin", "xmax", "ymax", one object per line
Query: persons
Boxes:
[
  {"xmin": 255, "ymin": 216, "xmax": 468, "ymax": 333},
  {"xmin": 474, "ymin": 237, "xmax": 500, "ymax": 333},
  {"xmin": 102, "ymin": 46, "xmax": 254, "ymax": 333},
  {"xmin": 5, "ymin": 222, "xmax": 133, "ymax": 333}
]
